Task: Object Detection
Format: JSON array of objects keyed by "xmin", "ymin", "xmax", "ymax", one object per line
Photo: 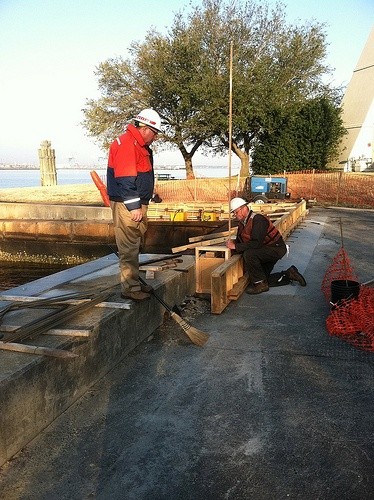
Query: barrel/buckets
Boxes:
[{"xmin": 331, "ymin": 280, "xmax": 360, "ymax": 310}]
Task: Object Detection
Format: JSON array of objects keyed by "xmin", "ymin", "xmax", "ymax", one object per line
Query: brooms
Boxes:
[{"xmin": 107, "ymin": 243, "xmax": 208, "ymax": 346}]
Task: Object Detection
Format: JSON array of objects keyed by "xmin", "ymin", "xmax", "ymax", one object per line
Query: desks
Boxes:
[{"xmin": 155, "ymin": 174, "xmax": 171, "ymax": 181}]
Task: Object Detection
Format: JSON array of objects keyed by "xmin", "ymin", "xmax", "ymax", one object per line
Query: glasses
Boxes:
[{"xmin": 148, "ymin": 127, "xmax": 158, "ymax": 136}]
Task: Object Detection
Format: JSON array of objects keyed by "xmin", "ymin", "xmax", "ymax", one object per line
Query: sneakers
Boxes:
[
  {"xmin": 246, "ymin": 281, "xmax": 268, "ymax": 294},
  {"xmin": 287, "ymin": 265, "xmax": 306, "ymax": 286},
  {"xmin": 140, "ymin": 285, "xmax": 153, "ymax": 293},
  {"xmin": 122, "ymin": 289, "xmax": 151, "ymax": 302}
]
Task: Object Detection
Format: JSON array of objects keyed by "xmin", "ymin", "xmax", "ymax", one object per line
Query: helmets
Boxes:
[
  {"xmin": 230, "ymin": 198, "xmax": 248, "ymax": 213},
  {"xmin": 132, "ymin": 109, "xmax": 162, "ymax": 133}
]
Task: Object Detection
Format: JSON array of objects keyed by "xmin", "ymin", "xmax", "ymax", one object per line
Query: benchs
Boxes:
[{"xmin": 155, "ymin": 176, "xmax": 175, "ymax": 179}]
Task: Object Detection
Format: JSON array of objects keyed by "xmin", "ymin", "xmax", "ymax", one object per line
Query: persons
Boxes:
[
  {"xmin": 226, "ymin": 197, "xmax": 307, "ymax": 295},
  {"xmin": 107, "ymin": 108, "xmax": 163, "ymax": 302}
]
